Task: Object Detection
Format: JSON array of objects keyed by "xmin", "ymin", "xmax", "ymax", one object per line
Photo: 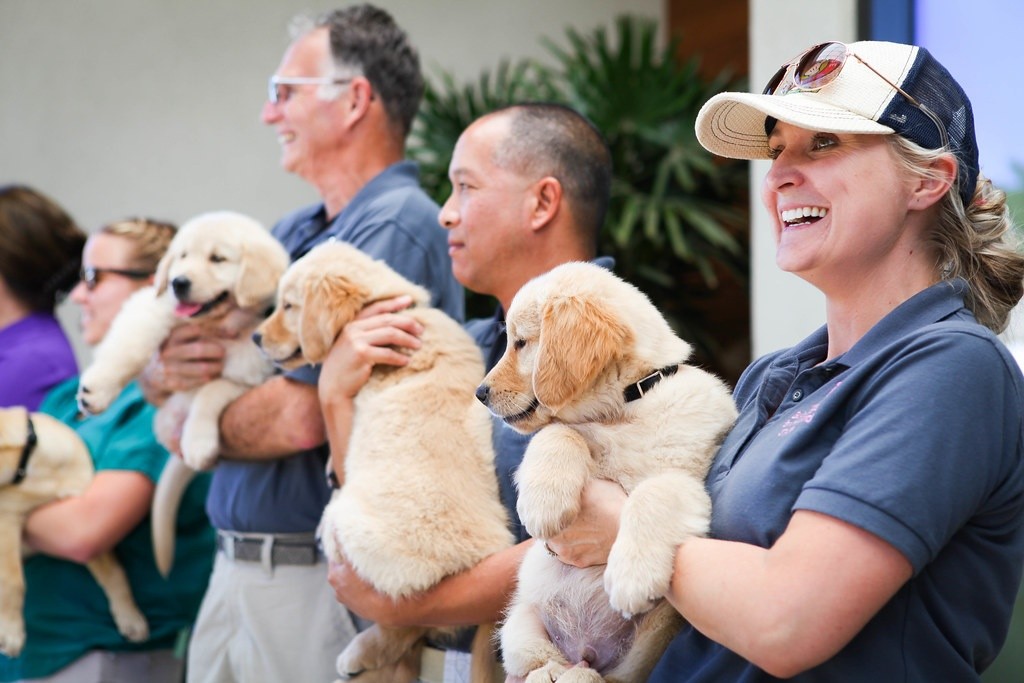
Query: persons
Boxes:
[
  {"xmin": 546, "ymin": 41, "xmax": 1024, "ymax": 683},
  {"xmin": 0, "ymin": 186, "xmax": 180, "ymax": 683},
  {"xmin": 318, "ymin": 105, "xmax": 618, "ymax": 683},
  {"xmin": 140, "ymin": 5, "xmax": 465, "ymax": 683}
]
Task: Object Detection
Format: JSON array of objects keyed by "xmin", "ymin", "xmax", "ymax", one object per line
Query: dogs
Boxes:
[
  {"xmin": 250, "ymin": 237, "xmax": 517, "ymax": 680},
  {"xmin": 0, "ymin": 405, "xmax": 148, "ymax": 657},
  {"xmin": 477, "ymin": 259, "xmax": 736, "ymax": 683},
  {"xmin": 77, "ymin": 211, "xmax": 293, "ymax": 575}
]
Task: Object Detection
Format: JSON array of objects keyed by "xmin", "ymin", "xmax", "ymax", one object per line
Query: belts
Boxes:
[
  {"xmin": 216, "ymin": 535, "xmax": 320, "ymax": 564},
  {"xmin": 415, "ymin": 626, "xmax": 501, "ymax": 659}
]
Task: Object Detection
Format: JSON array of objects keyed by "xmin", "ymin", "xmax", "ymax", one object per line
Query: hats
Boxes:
[{"xmin": 693, "ymin": 41, "xmax": 980, "ymax": 211}]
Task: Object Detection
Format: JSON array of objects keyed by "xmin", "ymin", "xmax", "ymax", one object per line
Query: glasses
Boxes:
[
  {"xmin": 81, "ymin": 267, "xmax": 151, "ymax": 290},
  {"xmin": 760, "ymin": 41, "xmax": 947, "ymax": 149},
  {"xmin": 269, "ymin": 74, "xmax": 376, "ymax": 106}
]
